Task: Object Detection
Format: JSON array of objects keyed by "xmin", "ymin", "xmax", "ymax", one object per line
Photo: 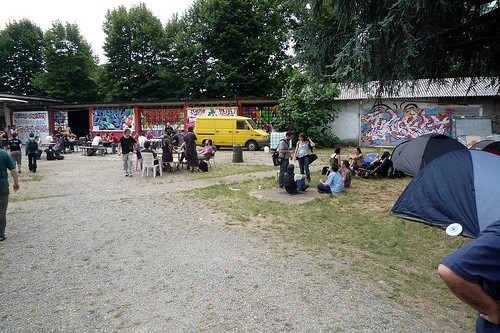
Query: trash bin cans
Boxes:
[
  {"xmin": 232, "ymin": 144, "xmax": 244, "ymax": 163},
  {"xmin": 270, "ymin": 132, "xmax": 292, "ymax": 152}
]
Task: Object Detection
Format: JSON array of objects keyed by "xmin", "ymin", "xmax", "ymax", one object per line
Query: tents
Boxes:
[
  {"xmin": 389, "ymin": 147, "xmax": 499, "ymax": 238},
  {"xmin": 388, "ymin": 133, "xmax": 468, "ymax": 178}
]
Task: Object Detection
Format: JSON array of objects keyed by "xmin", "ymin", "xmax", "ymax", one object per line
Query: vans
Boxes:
[{"xmin": 193, "ymin": 116, "xmax": 269, "ymax": 151}]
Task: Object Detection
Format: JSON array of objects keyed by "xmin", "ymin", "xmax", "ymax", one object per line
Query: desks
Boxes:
[
  {"xmin": 134, "ymin": 148, "xmax": 203, "ymax": 171},
  {"xmin": 87, "ymin": 140, "xmax": 116, "ymax": 156},
  {"xmin": 41, "ymin": 142, "xmax": 57, "ymax": 160}
]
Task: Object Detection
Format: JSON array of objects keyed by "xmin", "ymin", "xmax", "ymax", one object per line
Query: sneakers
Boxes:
[
  {"xmin": 0, "ymin": 235, "xmax": 6, "ymax": 241},
  {"xmin": 277, "ymin": 178, "xmax": 326, "ymax": 194},
  {"xmin": 123, "ymin": 163, "xmax": 195, "ymax": 177}
]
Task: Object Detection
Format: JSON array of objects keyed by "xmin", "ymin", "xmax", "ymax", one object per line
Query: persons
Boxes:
[
  {"xmin": 349, "ymin": 151, "xmax": 390, "ymax": 171},
  {"xmin": 0, "ymin": 127, "xmax": 9, "ymax": 152},
  {"xmin": 336, "ymin": 160, "xmax": 352, "ymax": 188},
  {"xmin": 294, "ymin": 132, "xmax": 315, "ymax": 182},
  {"xmin": 347, "ymin": 147, "xmax": 364, "ymax": 172},
  {"xmin": 283, "ymin": 164, "xmax": 309, "ymax": 194},
  {"xmin": 117, "ymin": 129, "xmax": 136, "ymax": 177},
  {"xmin": 316, "ymin": 163, "xmax": 344, "ymax": 194},
  {"xmin": 8, "ymin": 132, "xmax": 22, "ymax": 173},
  {"xmin": 0, "ymin": 149, "xmax": 20, "ymax": 242},
  {"xmin": 436, "ymin": 220, "xmax": 500, "ymax": 333},
  {"xmin": 278, "ymin": 132, "xmax": 296, "ymax": 188},
  {"xmin": 329, "ymin": 147, "xmax": 341, "ymax": 169},
  {"xmin": 133, "ymin": 122, "xmax": 213, "ymax": 177},
  {"xmin": 46, "ymin": 122, "xmax": 133, "ymax": 160},
  {"xmin": 24, "ymin": 133, "xmax": 38, "ymax": 173},
  {"xmin": 35, "ymin": 135, "xmax": 43, "ymax": 160},
  {"xmin": 9, "ymin": 125, "xmax": 17, "ymax": 139}
]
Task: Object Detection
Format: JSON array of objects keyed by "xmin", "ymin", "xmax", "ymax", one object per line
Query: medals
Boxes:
[{"xmin": 468, "ymin": 140, "xmax": 500, "ymax": 158}]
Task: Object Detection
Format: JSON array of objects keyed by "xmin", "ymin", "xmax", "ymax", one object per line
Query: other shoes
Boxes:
[
  {"xmin": 61, "ymin": 147, "xmax": 74, "ymax": 153},
  {"xmin": 29, "ymin": 168, "xmax": 36, "ymax": 173},
  {"xmin": 36, "ymin": 157, "xmax": 40, "ymax": 160},
  {"xmin": 55, "ymin": 154, "xmax": 59, "ymax": 158},
  {"xmin": 17, "ymin": 170, "xmax": 22, "ymax": 173}
]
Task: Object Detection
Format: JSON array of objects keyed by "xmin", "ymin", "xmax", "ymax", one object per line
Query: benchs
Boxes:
[{"xmin": 78, "ymin": 145, "xmax": 107, "ymax": 157}]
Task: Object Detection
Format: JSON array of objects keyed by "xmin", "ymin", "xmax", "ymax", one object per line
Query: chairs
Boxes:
[{"xmin": 140, "ymin": 152, "xmax": 162, "ymax": 178}]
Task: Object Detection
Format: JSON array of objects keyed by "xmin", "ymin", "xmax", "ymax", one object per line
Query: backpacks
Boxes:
[{"xmin": 198, "ymin": 161, "xmax": 208, "ymax": 173}]
